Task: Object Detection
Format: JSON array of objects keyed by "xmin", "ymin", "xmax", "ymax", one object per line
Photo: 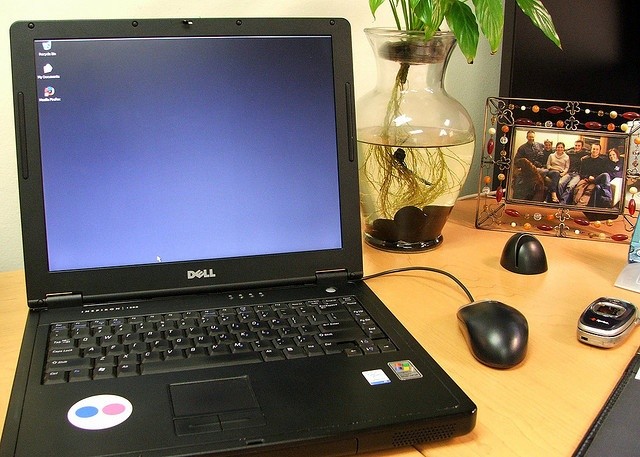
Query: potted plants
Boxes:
[{"xmin": 356, "ymin": 0, "xmax": 565, "ymax": 253}]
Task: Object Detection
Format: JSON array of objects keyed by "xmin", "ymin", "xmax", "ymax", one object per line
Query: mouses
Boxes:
[{"xmin": 460, "ymin": 294, "xmax": 530, "ymax": 367}]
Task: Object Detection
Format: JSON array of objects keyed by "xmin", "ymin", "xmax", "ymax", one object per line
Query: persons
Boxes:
[{"xmin": 514, "ymin": 127, "xmax": 623, "ymax": 210}]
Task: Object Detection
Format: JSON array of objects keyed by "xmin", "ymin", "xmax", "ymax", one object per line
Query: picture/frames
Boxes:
[{"xmin": 474, "ymin": 98, "xmax": 639, "ymax": 244}]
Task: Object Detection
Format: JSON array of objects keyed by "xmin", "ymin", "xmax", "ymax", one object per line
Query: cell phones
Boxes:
[{"xmin": 576, "ymin": 297, "xmax": 640, "ymax": 351}]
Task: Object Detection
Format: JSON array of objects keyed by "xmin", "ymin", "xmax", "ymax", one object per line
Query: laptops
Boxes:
[{"xmin": 4, "ymin": 16, "xmax": 479, "ymax": 455}]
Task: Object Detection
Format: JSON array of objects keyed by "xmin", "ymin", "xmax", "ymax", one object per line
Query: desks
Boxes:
[{"xmin": 0, "ymin": 195, "xmax": 640, "ymax": 455}]
